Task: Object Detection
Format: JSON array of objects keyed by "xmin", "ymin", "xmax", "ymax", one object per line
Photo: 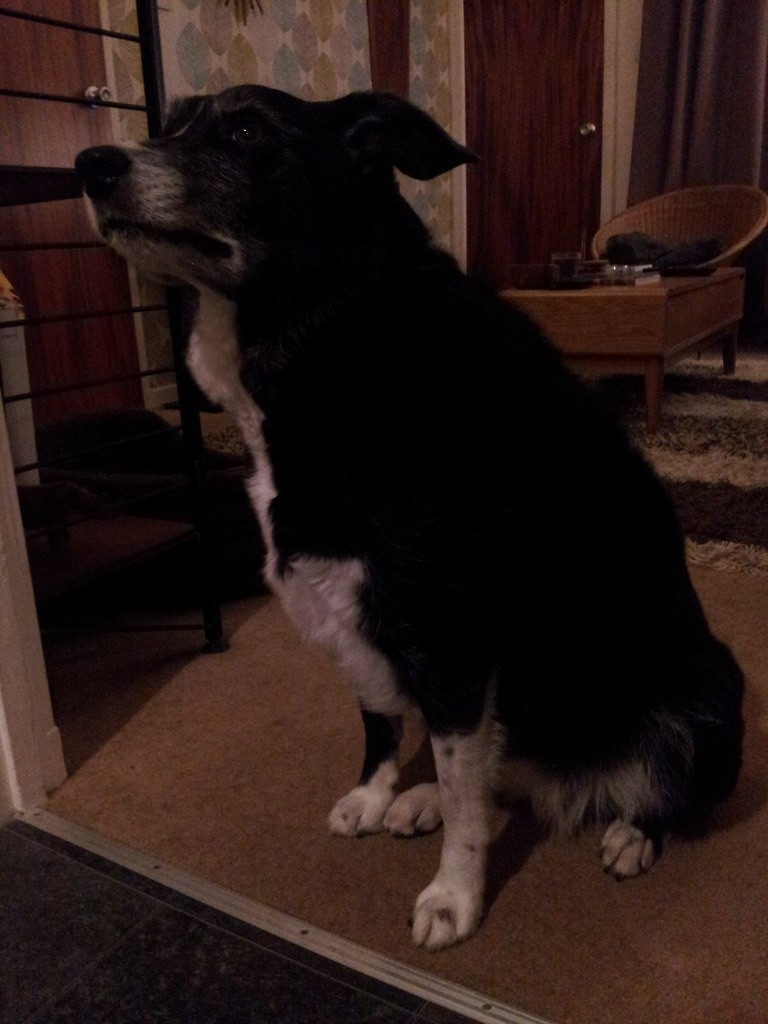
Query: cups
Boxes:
[{"xmin": 550, "ymin": 253, "xmax": 581, "ymax": 277}]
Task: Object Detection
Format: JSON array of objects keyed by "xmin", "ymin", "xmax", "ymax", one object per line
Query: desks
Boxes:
[{"xmin": 499, "ymin": 267, "xmax": 747, "ymax": 435}]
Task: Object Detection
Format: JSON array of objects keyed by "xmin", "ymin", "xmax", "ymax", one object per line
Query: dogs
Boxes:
[{"xmin": 70, "ymin": 81, "xmax": 748, "ymax": 952}]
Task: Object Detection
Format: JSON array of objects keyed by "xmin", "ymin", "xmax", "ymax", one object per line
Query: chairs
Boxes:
[{"xmin": 590, "ymin": 184, "xmax": 768, "ymax": 362}]
